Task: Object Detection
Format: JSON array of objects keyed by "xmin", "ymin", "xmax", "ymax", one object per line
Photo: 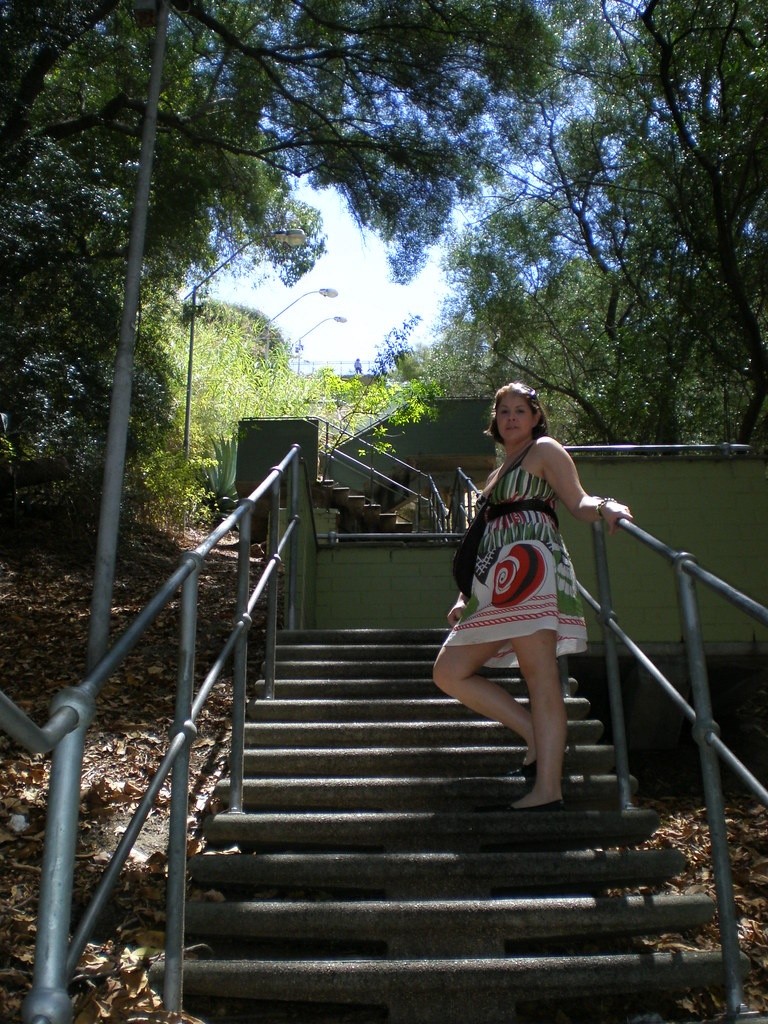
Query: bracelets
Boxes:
[{"xmin": 596, "ymin": 497, "xmax": 618, "ymax": 520}]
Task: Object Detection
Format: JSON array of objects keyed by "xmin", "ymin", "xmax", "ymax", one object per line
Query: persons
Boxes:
[{"xmin": 432, "ymin": 382, "xmax": 633, "ymax": 814}]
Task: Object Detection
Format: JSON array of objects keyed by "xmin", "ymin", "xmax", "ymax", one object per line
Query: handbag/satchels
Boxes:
[{"xmin": 452, "ymin": 501, "xmax": 493, "ymax": 598}]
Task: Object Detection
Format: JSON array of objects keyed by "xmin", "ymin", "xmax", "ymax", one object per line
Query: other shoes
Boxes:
[
  {"xmin": 507, "ymin": 758, "xmax": 539, "ymax": 777},
  {"xmin": 502, "ymin": 798, "xmax": 565, "ymax": 813}
]
